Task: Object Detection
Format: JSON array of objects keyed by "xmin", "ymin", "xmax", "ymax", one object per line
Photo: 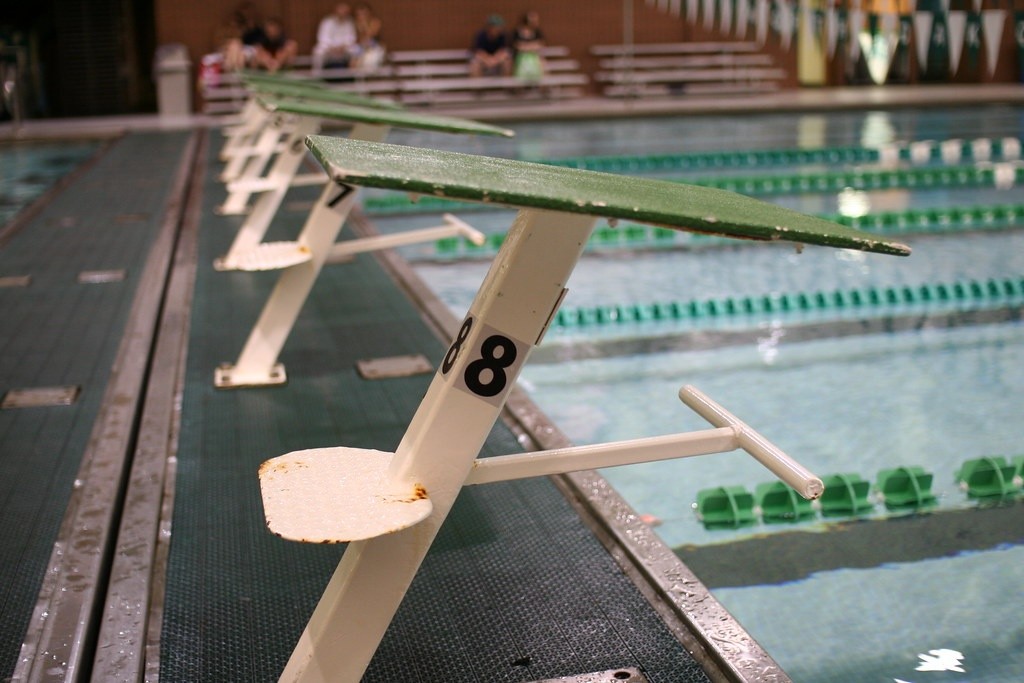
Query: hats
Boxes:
[{"xmin": 487, "ymin": 14, "xmax": 507, "ymax": 28}]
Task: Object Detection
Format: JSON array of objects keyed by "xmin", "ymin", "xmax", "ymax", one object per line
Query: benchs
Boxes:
[{"xmin": 199, "ymin": 41, "xmax": 788, "ymax": 115}]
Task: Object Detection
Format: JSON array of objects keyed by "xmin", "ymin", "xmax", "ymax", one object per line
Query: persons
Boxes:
[
  {"xmin": 0, "ymin": 30, "xmax": 28, "ymax": 123},
  {"xmin": 467, "ymin": 10, "xmax": 545, "ymax": 78},
  {"xmin": 203, "ymin": 0, "xmax": 298, "ymax": 77},
  {"xmin": 312, "ymin": 0, "xmax": 385, "ymax": 83}
]
